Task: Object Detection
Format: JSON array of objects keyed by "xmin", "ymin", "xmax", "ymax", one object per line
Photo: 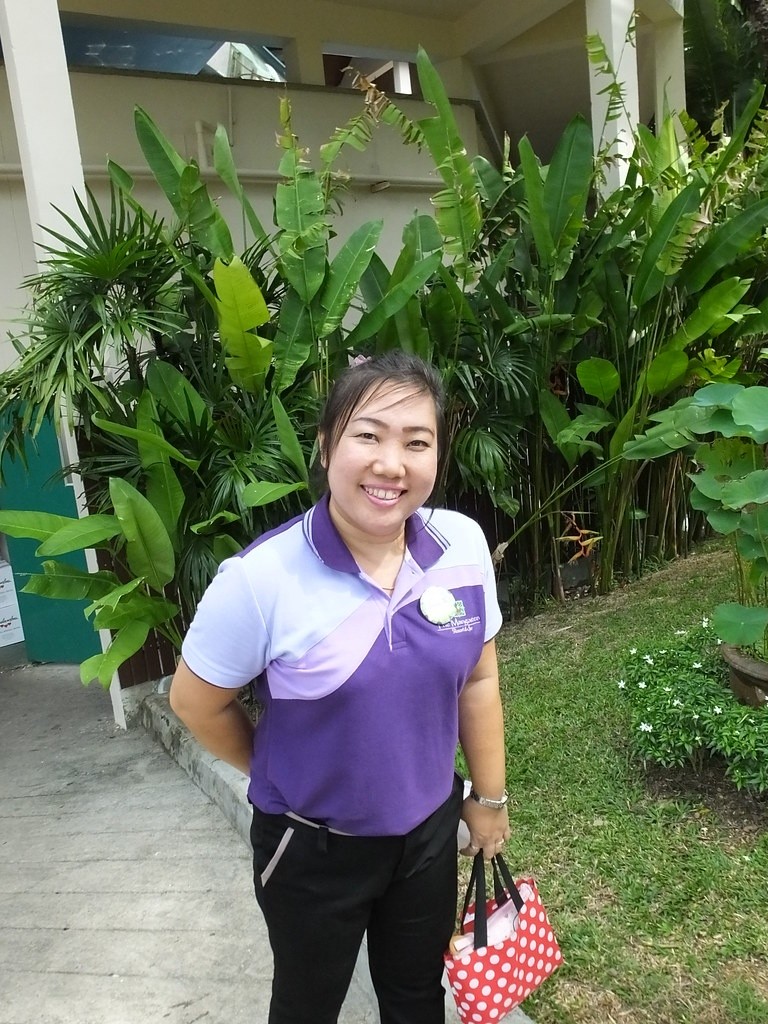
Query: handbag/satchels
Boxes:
[{"xmin": 445, "ymin": 845, "xmax": 563, "ymax": 1024}]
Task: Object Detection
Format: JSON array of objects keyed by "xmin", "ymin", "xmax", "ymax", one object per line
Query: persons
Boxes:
[{"xmin": 170, "ymin": 348, "xmax": 512, "ymax": 1024}]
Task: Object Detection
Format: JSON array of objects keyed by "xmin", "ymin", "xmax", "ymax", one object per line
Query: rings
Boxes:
[
  {"xmin": 498, "ymin": 838, "xmax": 504, "ymax": 845},
  {"xmin": 470, "ymin": 843, "xmax": 479, "ymax": 849}
]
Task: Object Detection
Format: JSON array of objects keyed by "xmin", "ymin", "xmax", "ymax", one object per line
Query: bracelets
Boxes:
[{"xmin": 469, "ymin": 787, "xmax": 509, "ymax": 808}]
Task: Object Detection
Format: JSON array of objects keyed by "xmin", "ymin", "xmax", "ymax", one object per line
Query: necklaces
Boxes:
[{"xmin": 383, "ymin": 588, "xmax": 394, "ymax": 590}]
